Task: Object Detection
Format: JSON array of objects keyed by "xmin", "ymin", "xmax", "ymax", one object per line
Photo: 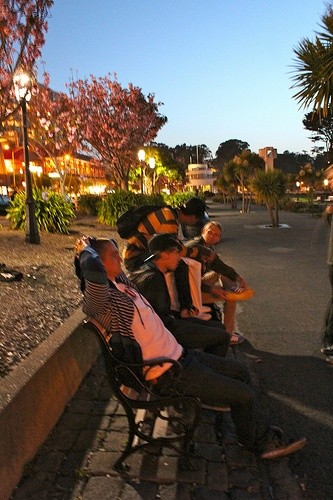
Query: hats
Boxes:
[
  {"xmin": 184, "ymin": 198, "xmax": 210, "ymax": 221},
  {"xmin": 140, "ymin": 233, "xmax": 181, "ymax": 261}
]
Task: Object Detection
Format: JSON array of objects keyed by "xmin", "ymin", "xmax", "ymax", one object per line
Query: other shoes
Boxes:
[
  {"xmin": 180, "ymin": 305, "xmax": 205, "ymax": 318},
  {"xmin": 200, "ymin": 404, "xmax": 231, "ymax": 412},
  {"xmin": 325, "ymin": 355, "xmax": 333, "ymax": 364},
  {"xmin": 260, "ymin": 437, "xmax": 307, "ymax": 459}
]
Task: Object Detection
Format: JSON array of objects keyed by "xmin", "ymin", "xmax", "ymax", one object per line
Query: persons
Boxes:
[
  {"xmin": 322, "ymin": 205, "xmax": 333, "ymax": 366},
  {"xmin": 74, "ymin": 236, "xmax": 306, "ymax": 459},
  {"xmin": 124, "ymin": 198, "xmax": 256, "ymax": 357}
]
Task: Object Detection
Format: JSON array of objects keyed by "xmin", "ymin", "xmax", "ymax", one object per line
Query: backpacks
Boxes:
[{"xmin": 115, "ymin": 205, "xmax": 174, "ymax": 239}]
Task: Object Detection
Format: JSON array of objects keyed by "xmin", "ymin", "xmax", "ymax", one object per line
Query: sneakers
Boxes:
[
  {"xmin": 229, "ymin": 334, "xmax": 246, "ymax": 345},
  {"xmin": 224, "ymin": 287, "xmax": 255, "ymax": 302}
]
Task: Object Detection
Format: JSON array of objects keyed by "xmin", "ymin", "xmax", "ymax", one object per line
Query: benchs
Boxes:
[{"xmin": 83, "ymin": 302, "xmax": 232, "ymax": 471}]
